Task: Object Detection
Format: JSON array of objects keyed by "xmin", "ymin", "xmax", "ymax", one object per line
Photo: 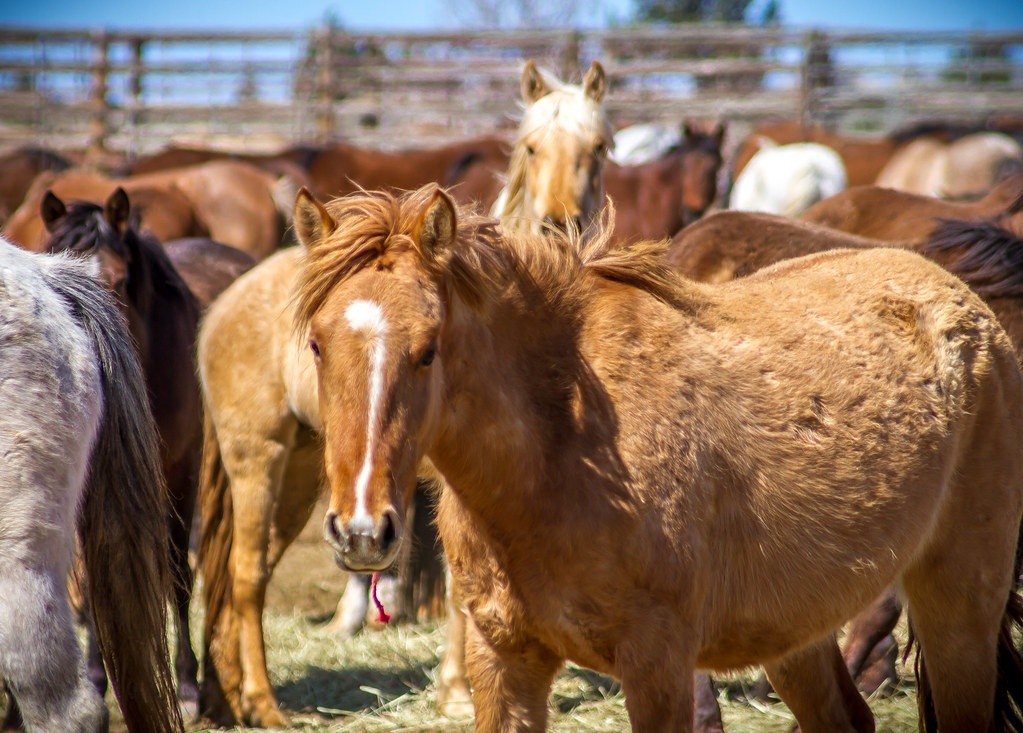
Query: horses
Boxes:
[{"xmin": 0, "ymin": 57, "xmax": 1023, "ymax": 733}]
